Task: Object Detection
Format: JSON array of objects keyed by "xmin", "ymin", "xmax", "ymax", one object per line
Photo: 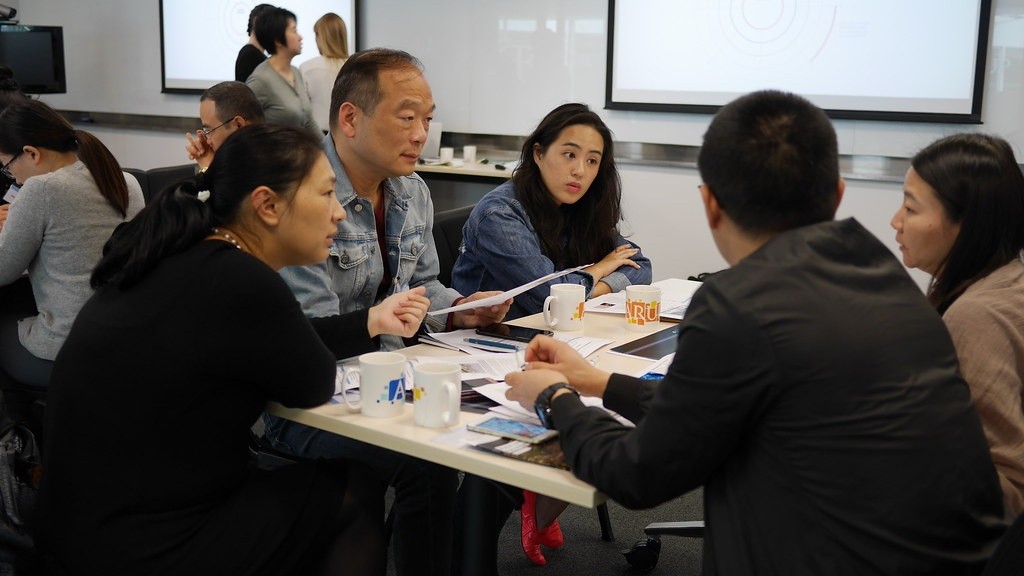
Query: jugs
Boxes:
[{"xmin": 418, "ymin": 122, "xmax": 442, "ymax": 159}]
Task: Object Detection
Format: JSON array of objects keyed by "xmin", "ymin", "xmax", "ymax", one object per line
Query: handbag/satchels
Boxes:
[{"xmin": 0, "ymin": 421, "xmax": 42, "ymax": 526}]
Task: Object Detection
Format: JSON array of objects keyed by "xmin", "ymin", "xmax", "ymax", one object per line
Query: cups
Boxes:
[
  {"xmin": 625, "ymin": 284, "xmax": 662, "ymax": 333},
  {"xmin": 412, "ymin": 361, "xmax": 462, "ymax": 428},
  {"xmin": 439, "ymin": 147, "xmax": 453, "ymax": 163},
  {"xmin": 463, "ymin": 145, "xmax": 477, "ymax": 162},
  {"xmin": 542, "ymin": 283, "xmax": 586, "ymax": 332},
  {"xmin": 341, "ymin": 351, "xmax": 406, "ymax": 419}
]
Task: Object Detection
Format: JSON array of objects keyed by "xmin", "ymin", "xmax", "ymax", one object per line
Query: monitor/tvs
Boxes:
[{"xmin": 0, "ymin": 24, "xmax": 67, "ymax": 94}]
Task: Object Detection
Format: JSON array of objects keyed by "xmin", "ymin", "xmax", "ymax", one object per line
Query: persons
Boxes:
[
  {"xmin": 451, "ymin": 102, "xmax": 652, "ymax": 564},
  {"xmin": 235, "ymin": 4, "xmax": 275, "ymax": 83},
  {"xmin": 247, "ymin": 8, "xmax": 325, "ymax": 141},
  {"xmin": 891, "ymin": 134, "xmax": 1024, "ymax": 524},
  {"xmin": 505, "ymin": 90, "xmax": 1008, "ymax": 576},
  {"xmin": 0, "ymin": 100, "xmax": 146, "ymax": 421},
  {"xmin": 300, "ymin": 13, "xmax": 350, "ymax": 137},
  {"xmin": 261, "ymin": 48, "xmax": 519, "ymax": 576},
  {"xmin": 36, "ymin": 122, "xmax": 388, "ymax": 576},
  {"xmin": 186, "ymin": 82, "xmax": 261, "ymax": 203}
]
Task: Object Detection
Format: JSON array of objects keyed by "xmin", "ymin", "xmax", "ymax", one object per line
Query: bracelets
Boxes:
[{"xmin": 199, "ymin": 168, "xmax": 208, "ymax": 173}]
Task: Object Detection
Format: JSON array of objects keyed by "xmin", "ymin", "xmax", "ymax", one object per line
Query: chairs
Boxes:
[
  {"xmin": 435, "ymin": 202, "xmax": 623, "ymax": 538},
  {"xmin": 121, "ymin": 165, "xmax": 201, "ymax": 199}
]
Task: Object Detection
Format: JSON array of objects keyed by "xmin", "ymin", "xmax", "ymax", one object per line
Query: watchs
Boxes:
[{"xmin": 534, "ymin": 382, "xmax": 581, "ymax": 430}]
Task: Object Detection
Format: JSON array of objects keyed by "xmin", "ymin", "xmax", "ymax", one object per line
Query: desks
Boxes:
[
  {"xmin": 414, "ymin": 157, "xmax": 521, "ymax": 184},
  {"xmin": 265, "ymin": 280, "xmax": 703, "ymax": 540}
]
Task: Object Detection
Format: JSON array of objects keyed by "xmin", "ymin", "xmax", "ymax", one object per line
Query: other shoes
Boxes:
[
  {"xmin": 518, "ymin": 488, "xmax": 546, "ymax": 566},
  {"xmin": 540, "ymin": 517, "xmax": 563, "ymax": 548}
]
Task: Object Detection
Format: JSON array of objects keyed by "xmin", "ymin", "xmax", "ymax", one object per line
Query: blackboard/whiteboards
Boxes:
[{"xmin": 0, "ymin": 0, "xmax": 1024, "ymax": 186}]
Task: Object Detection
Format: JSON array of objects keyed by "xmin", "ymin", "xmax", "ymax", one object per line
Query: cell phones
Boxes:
[
  {"xmin": 466, "ymin": 412, "xmax": 560, "ymax": 443},
  {"xmin": 476, "ymin": 323, "xmax": 555, "ymax": 343}
]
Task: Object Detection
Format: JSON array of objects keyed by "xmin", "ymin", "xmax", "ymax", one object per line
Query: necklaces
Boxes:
[{"xmin": 212, "ymin": 228, "xmax": 242, "ymax": 250}]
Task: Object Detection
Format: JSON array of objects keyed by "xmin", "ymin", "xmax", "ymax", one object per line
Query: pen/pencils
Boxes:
[
  {"xmin": 417, "ymin": 157, "xmax": 425, "ymax": 164},
  {"xmin": 463, "ymin": 338, "xmax": 518, "ymax": 350},
  {"xmin": 494, "ymin": 164, "xmax": 507, "ymax": 171}
]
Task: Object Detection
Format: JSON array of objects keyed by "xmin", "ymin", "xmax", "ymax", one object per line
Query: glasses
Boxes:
[
  {"xmin": 0, "ymin": 146, "xmax": 22, "ymax": 180},
  {"xmin": 202, "ymin": 116, "xmax": 246, "ymax": 140}
]
